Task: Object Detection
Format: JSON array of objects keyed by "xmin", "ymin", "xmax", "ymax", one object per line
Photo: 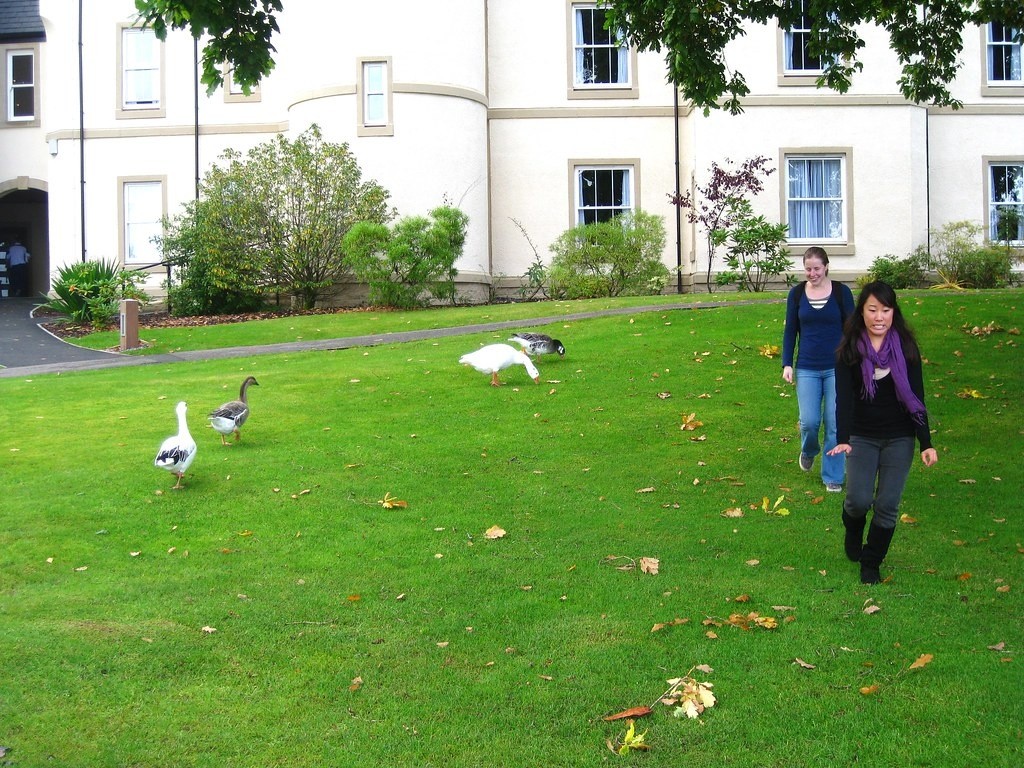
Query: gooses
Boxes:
[{"xmin": 459, "ymin": 343, "xmax": 540, "ymax": 386}]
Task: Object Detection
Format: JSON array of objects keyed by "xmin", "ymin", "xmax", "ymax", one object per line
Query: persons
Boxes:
[
  {"xmin": 7, "ymin": 239, "xmax": 30, "ymax": 297},
  {"xmin": 827, "ymin": 281, "xmax": 938, "ymax": 584},
  {"xmin": 781, "ymin": 247, "xmax": 856, "ymax": 492}
]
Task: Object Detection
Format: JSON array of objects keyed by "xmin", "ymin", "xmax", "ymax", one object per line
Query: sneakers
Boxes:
[
  {"xmin": 825, "ymin": 483, "xmax": 842, "ymax": 493},
  {"xmin": 799, "ymin": 449, "xmax": 814, "ymax": 471}
]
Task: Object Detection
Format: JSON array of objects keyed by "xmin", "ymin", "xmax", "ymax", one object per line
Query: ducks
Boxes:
[
  {"xmin": 155, "ymin": 401, "xmax": 197, "ymax": 490},
  {"xmin": 207, "ymin": 376, "xmax": 259, "ymax": 446},
  {"xmin": 508, "ymin": 332, "xmax": 565, "ymax": 361}
]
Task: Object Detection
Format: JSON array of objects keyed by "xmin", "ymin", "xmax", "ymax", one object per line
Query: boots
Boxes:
[
  {"xmin": 842, "ymin": 499, "xmax": 866, "ymax": 562},
  {"xmin": 861, "ymin": 519, "xmax": 896, "ymax": 585}
]
